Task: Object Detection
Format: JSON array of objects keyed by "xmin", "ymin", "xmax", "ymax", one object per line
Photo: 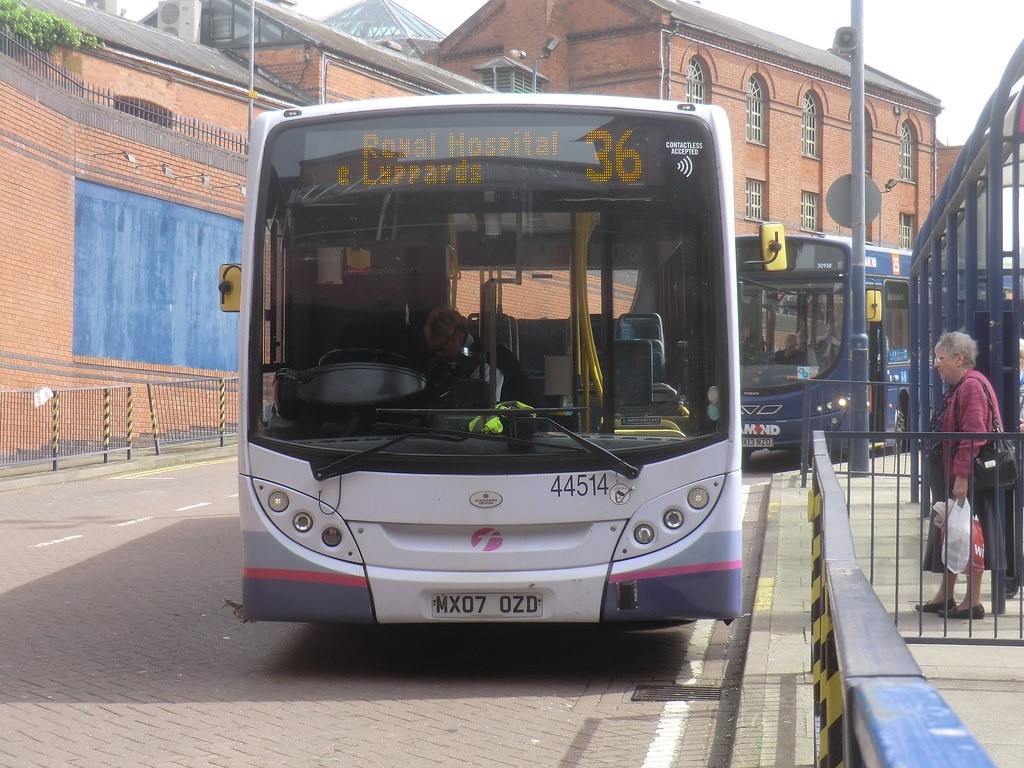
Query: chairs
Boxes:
[
  {"xmin": 565, "ymin": 312, "xmax": 667, "ymax": 387},
  {"xmin": 466, "ymin": 311, "xmax": 519, "ymax": 361}
]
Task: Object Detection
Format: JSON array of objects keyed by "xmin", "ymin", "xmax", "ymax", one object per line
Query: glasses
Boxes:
[
  {"xmin": 934, "ymin": 354, "xmax": 952, "ymax": 364},
  {"xmin": 431, "ymin": 333, "xmax": 455, "ymax": 356}
]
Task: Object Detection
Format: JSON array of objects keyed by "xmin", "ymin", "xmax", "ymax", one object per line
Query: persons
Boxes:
[
  {"xmin": 740, "ymin": 321, "xmax": 761, "ymax": 358},
  {"xmin": 915, "ymin": 331, "xmax": 1002, "ymax": 620},
  {"xmin": 421, "ymin": 307, "xmax": 540, "ymax": 424},
  {"xmin": 773, "ymin": 333, "xmax": 807, "ymax": 365},
  {"xmin": 1018, "ymin": 337, "xmax": 1024, "ymax": 447}
]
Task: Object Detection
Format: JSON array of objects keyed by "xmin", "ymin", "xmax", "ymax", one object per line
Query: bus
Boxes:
[
  {"xmin": 218, "ymin": 93, "xmax": 785, "ymax": 626},
  {"xmin": 728, "ymin": 231, "xmax": 1014, "ymax": 470}
]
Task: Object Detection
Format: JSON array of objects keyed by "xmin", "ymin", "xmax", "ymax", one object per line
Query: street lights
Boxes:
[
  {"xmin": 509, "ymin": 36, "xmax": 561, "ymax": 92},
  {"xmin": 248, "ymin": 1, "xmax": 295, "ymax": 155}
]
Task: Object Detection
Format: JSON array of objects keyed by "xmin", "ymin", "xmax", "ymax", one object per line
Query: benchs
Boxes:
[{"xmin": 515, "ymin": 318, "xmax": 619, "ymax": 410}]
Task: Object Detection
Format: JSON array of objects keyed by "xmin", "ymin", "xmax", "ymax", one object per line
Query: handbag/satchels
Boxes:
[
  {"xmin": 942, "ymin": 495, "xmax": 971, "ymax": 576},
  {"xmin": 939, "ymin": 515, "xmax": 985, "ymax": 577},
  {"xmin": 954, "ymin": 376, "xmax": 1019, "ymax": 496}
]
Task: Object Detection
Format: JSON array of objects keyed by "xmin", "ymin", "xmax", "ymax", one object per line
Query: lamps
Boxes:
[
  {"xmin": 92, "ymin": 151, "xmax": 136, "ymax": 165},
  {"xmin": 175, "ymin": 173, "xmax": 210, "ymax": 186},
  {"xmin": 211, "ymin": 184, "xmax": 246, "ymax": 196},
  {"xmin": 136, "ymin": 164, "xmax": 173, "ymax": 177}
]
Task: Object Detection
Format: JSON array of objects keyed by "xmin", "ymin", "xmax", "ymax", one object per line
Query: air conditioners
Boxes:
[{"xmin": 158, "ymin": 0, "xmax": 202, "ymax": 45}]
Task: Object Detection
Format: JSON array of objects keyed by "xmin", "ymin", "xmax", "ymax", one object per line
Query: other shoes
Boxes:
[
  {"xmin": 915, "ymin": 599, "xmax": 956, "ymax": 613},
  {"xmin": 937, "ymin": 603, "xmax": 985, "ymax": 619}
]
